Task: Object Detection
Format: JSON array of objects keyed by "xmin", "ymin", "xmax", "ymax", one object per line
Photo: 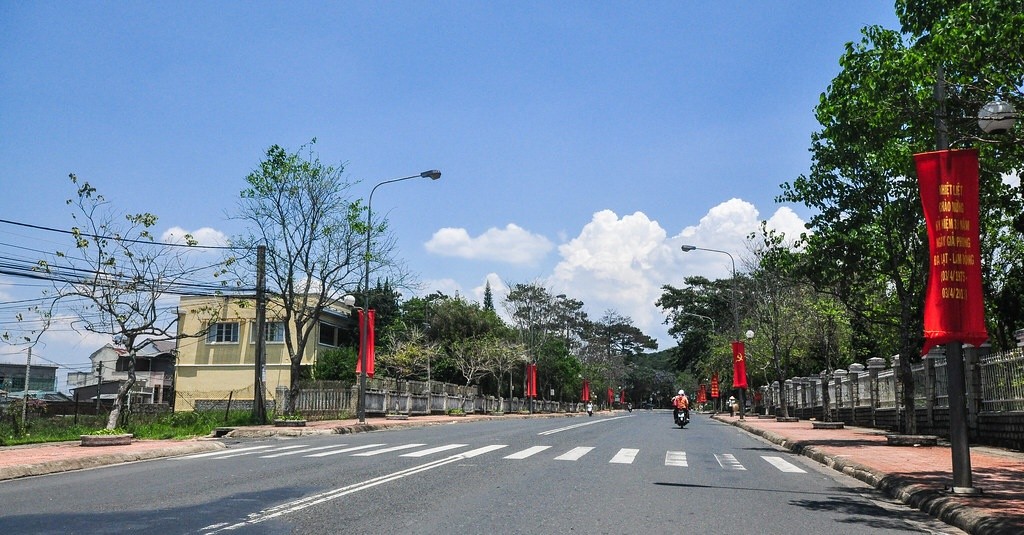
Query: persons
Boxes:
[
  {"xmin": 587, "ymin": 401, "xmax": 594, "ymax": 414},
  {"xmin": 671, "ymin": 390, "xmax": 691, "ymax": 424},
  {"xmin": 727, "ymin": 395, "xmax": 736, "ymax": 417}
]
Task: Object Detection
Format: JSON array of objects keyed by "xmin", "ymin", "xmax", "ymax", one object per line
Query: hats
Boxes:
[{"xmin": 729, "ymin": 396, "xmax": 736, "ymax": 399}]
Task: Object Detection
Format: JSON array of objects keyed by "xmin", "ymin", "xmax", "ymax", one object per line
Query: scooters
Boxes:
[
  {"xmin": 587, "ymin": 405, "xmax": 593, "ymax": 417},
  {"xmin": 628, "ymin": 407, "xmax": 632, "ymax": 413},
  {"xmin": 674, "ymin": 408, "xmax": 690, "ymax": 429}
]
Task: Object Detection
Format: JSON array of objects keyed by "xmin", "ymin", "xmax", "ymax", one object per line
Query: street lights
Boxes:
[
  {"xmin": 530, "ymin": 294, "xmax": 566, "ymax": 414},
  {"xmin": 344, "ymin": 169, "xmax": 441, "ymax": 425},
  {"xmin": 685, "ymin": 313, "xmax": 718, "ymax": 415},
  {"xmin": 681, "ymin": 245, "xmax": 755, "ymax": 422},
  {"xmin": 936, "ymin": 66, "xmax": 1017, "ymax": 498}
]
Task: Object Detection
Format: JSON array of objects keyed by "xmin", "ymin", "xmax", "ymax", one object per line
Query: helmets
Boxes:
[{"xmin": 678, "ymin": 390, "xmax": 684, "ymax": 395}]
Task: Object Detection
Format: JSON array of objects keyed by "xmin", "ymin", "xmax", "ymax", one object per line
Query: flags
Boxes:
[
  {"xmin": 527, "ymin": 365, "xmax": 537, "ymax": 397},
  {"xmin": 731, "ymin": 342, "xmax": 748, "ymax": 389},
  {"xmin": 697, "ymin": 384, "xmax": 707, "ymax": 403},
  {"xmin": 913, "ymin": 149, "xmax": 988, "ymax": 356},
  {"xmin": 582, "ymin": 380, "xmax": 589, "ymax": 401},
  {"xmin": 711, "ymin": 372, "xmax": 719, "ymax": 397},
  {"xmin": 355, "ymin": 310, "xmax": 375, "ymax": 379}
]
